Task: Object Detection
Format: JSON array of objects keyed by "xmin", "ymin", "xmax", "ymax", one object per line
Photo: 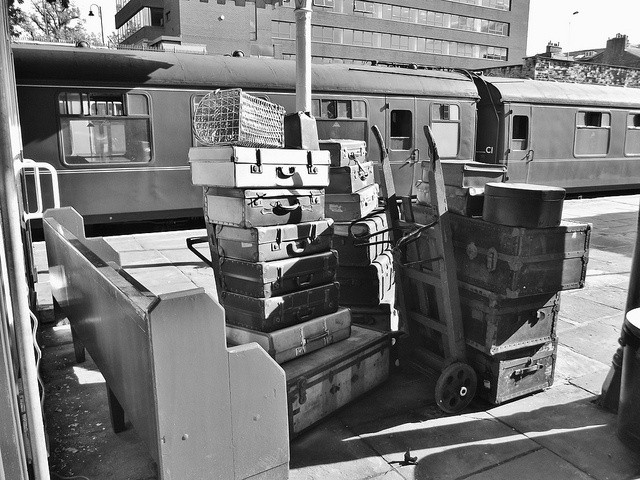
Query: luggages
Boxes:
[
  {"xmin": 399, "ymin": 264, "xmax": 560, "ymax": 356},
  {"xmin": 215, "ymin": 218, "xmax": 334, "ymax": 262},
  {"xmin": 397, "ymin": 316, "xmax": 557, "ymax": 406},
  {"xmin": 226, "ymin": 306, "xmax": 352, "ymax": 365},
  {"xmin": 325, "ymin": 161, "xmax": 375, "ymax": 193},
  {"xmin": 219, "ymin": 249, "xmax": 339, "ymax": 298},
  {"xmin": 220, "ymin": 281, "xmax": 338, "ymax": 333},
  {"xmin": 325, "ymin": 183, "xmax": 379, "ymax": 223},
  {"xmin": 203, "ymin": 187, "xmax": 325, "ymax": 230},
  {"xmin": 349, "ymin": 283, "xmax": 399, "ymax": 332},
  {"xmin": 319, "ymin": 139, "xmax": 367, "ymax": 169},
  {"xmin": 421, "ymin": 160, "xmax": 508, "ymax": 190},
  {"xmin": 333, "ymin": 207, "xmax": 392, "ymax": 268},
  {"xmin": 415, "ymin": 180, "xmax": 485, "ymax": 217},
  {"xmin": 188, "ymin": 146, "xmax": 331, "ymax": 188},
  {"xmin": 280, "ymin": 322, "xmax": 392, "ymax": 439},
  {"xmin": 338, "ymin": 246, "xmax": 395, "ymax": 306}
]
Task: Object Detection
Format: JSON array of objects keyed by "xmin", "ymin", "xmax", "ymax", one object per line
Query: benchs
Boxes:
[{"xmin": 40, "ymin": 202, "xmax": 292, "ymax": 480}]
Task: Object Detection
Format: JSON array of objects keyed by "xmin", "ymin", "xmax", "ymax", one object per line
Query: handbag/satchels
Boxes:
[{"xmin": 285, "ymin": 111, "xmax": 320, "ymax": 150}]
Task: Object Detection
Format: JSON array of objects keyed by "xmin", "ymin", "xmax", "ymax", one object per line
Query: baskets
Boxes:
[{"xmin": 192, "ymin": 89, "xmax": 287, "ymax": 148}]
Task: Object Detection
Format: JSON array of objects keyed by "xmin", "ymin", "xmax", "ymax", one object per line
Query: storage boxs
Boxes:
[
  {"xmin": 425, "ymin": 338, "xmax": 569, "ymax": 407},
  {"xmin": 404, "ymin": 283, "xmax": 565, "ymax": 356}
]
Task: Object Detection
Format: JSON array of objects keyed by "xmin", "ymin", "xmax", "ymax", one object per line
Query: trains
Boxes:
[{"xmin": 12, "ymin": 40, "xmax": 639, "ymax": 234}]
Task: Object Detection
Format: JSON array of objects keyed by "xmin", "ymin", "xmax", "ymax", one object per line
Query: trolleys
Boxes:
[{"xmin": 348, "ymin": 125, "xmax": 484, "ymax": 416}]
